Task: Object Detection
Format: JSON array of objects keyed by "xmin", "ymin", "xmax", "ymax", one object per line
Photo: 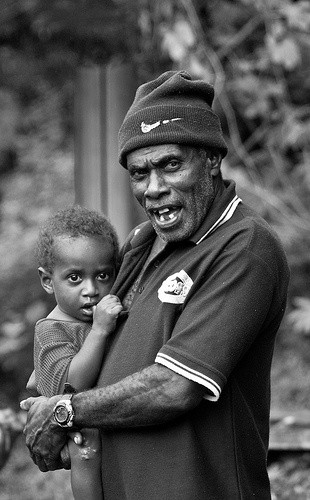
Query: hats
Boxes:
[{"xmin": 117, "ymin": 70, "xmax": 228, "ymax": 169}]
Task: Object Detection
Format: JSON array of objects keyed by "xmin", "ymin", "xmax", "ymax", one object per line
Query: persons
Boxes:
[
  {"xmin": 33, "ymin": 203, "xmax": 123, "ymax": 500},
  {"xmin": 19, "ymin": 70, "xmax": 289, "ymax": 500}
]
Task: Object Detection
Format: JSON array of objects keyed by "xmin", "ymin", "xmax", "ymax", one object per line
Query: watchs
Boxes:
[{"xmin": 53, "ymin": 393, "xmax": 75, "ymax": 427}]
